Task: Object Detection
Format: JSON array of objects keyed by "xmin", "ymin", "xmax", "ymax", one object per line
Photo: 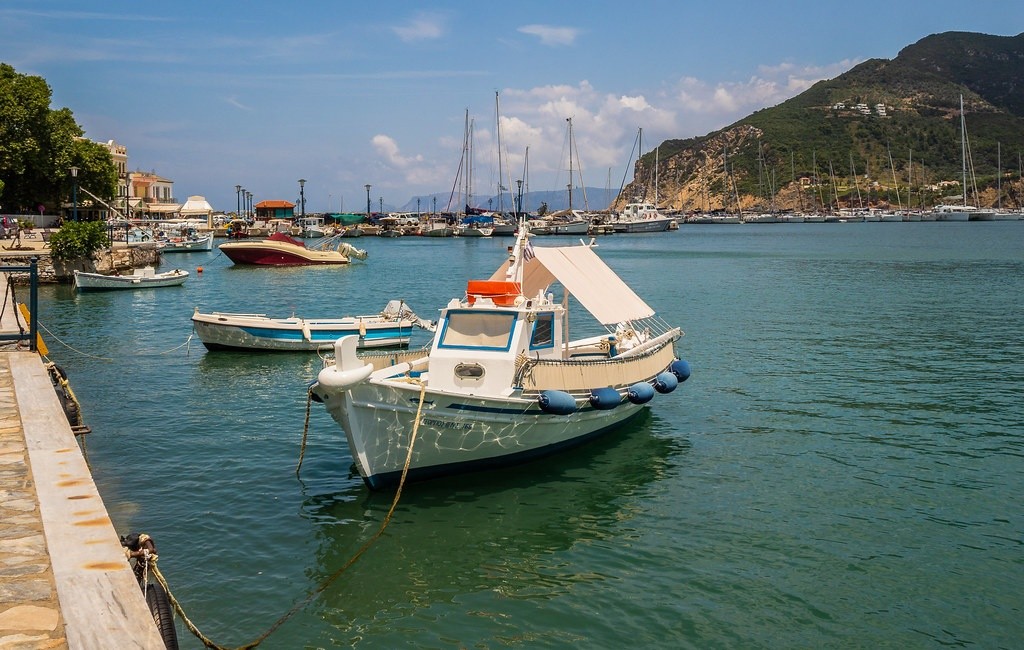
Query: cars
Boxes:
[
  {"xmin": 0, "ymin": 215, "xmax": 20, "ymax": 239},
  {"xmin": 368, "ymin": 213, "xmax": 392, "ymax": 226},
  {"xmin": 390, "ymin": 212, "xmax": 419, "ymax": 226},
  {"xmin": 508, "ymin": 211, "xmax": 543, "ymax": 222}
]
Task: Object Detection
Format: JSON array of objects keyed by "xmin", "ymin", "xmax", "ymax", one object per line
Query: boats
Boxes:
[
  {"xmin": 73, "ymin": 265, "xmax": 190, "ymax": 291},
  {"xmin": 191, "ymin": 305, "xmax": 419, "ymax": 354},
  {"xmin": 217, "ymin": 229, "xmax": 369, "ymax": 268},
  {"xmin": 305, "ymin": 212, "xmax": 692, "ymax": 496},
  {"xmin": 79, "ymin": 186, "xmax": 214, "ymax": 252}
]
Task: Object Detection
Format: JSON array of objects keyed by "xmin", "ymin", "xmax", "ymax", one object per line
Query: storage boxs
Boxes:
[
  {"xmin": 145, "ymin": 270, "xmax": 155, "ymax": 278},
  {"xmin": 134, "ymin": 269, "xmax": 145, "ymax": 278}
]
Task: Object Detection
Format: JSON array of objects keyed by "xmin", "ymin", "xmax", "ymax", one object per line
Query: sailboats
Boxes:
[{"xmin": 375, "ymin": 81, "xmax": 1023, "ymax": 239}]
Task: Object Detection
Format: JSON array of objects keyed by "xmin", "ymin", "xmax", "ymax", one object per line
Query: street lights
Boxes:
[
  {"xmin": 364, "ymin": 183, "xmax": 373, "ymax": 216},
  {"xmin": 566, "ymin": 183, "xmax": 573, "ymax": 208},
  {"xmin": 250, "ymin": 194, "xmax": 255, "ymax": 217},
  {"xmin": 516, "ymin": 178, "xmax": 525, "ymax": 212},
  {"xmin": 245, "ymin": 191, "xmax": 251, "ymax": 218},
  {"xmin": 122, "ymin": 171, "xmax": 132, "ymax": 244},
  {"xmin": 69, "ymin": 165, "xmax": 81, "ymax": 223},
  {"xmin": 416, "ymin": 197, "xmax": 421, "ymax": 213},
  {"xmin": 236, "ymin": 184, "xmax": 242, "ymax": 216},
  {"xmin": 377, "ymin": 196, "xmax": 384, "ymax": 213},
  {"xmin": 298, "ymin": 178, "xmax": 308, "ymax": 218},
  {"xmin": 433, "ymin": 196, "xmax": 437, "ymax": 213},
  {"xmin": 241, "ymin": 188, "xmax": 247, "ymax": 219}
]
